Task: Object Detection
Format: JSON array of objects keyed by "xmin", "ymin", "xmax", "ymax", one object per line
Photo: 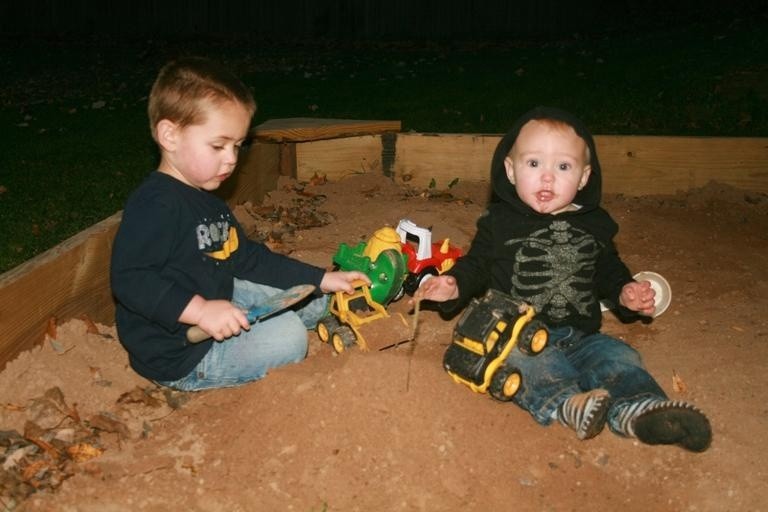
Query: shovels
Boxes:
[{"xmin": 187, "ymin": 284, "xmax": 316, "ymax": 343}]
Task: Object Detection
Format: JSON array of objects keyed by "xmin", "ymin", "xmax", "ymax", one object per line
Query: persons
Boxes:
[
  {"xmin": 407, "ymin": 105, "xmax": 713, "ymax": 454},
  {"xmin": 110, "ymin": 56, "xmax": 371, "ymax": 394}
]
